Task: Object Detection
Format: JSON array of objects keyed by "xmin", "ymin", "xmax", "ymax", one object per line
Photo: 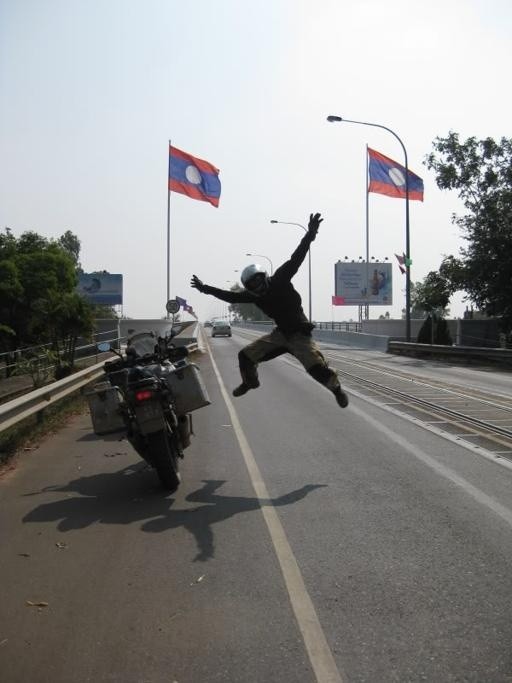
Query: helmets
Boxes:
[{"xmin": 241, "ymin": 264, "xmax": 271, "ymax": 297}]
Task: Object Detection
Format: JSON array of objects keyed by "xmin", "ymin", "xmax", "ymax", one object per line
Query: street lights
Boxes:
[
  {"xmin": 272, "ymin": 220, "xmax": 313, "ymax": 323},
  {"xmin": 328, "ymin": 114, "xmax": 411, "ymax": 343},
  {"xmin": 247, "ymin": 253, "xmax": 272, "ymax": 270}
]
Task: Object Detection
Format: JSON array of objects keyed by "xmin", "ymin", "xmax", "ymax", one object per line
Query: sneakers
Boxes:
[
  {"xmin": 233, "ymin": 380, "xmax": 260, "ymax": 396},
  {"xmin": 333, "ymin": 386, "xmax": 348, "ymax": 408}
]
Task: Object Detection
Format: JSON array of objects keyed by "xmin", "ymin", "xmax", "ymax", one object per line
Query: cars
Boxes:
[
  {"xmin": 204, "ymin": 320, "xmax": 214, "ymax": 327},
  {"xmin": 212, "ymin": 320, "xmax": 232, "ymax": 337}
]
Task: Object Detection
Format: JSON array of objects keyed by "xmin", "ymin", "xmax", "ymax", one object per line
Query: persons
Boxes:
[{"xmin": 190, "ymin": 213, "xmax": 349, "ymax": 409}]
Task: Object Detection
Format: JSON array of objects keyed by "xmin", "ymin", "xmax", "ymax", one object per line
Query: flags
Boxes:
[
  {"xmin": 168, "ymin": 145, "xmax": 221, "ymax": 209},
  {"xmin": 367, "ymin": 147, "xmax": 424, "ymax": 202}
]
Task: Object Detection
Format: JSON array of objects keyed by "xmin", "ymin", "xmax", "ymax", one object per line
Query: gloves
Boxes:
[
  {"xmin": 305, "ymin": 213, "xmax": 323, "ymax": 241},
  {"xmin": 190, "ymin": 275, "xmax": 208, "ymax": 292}
]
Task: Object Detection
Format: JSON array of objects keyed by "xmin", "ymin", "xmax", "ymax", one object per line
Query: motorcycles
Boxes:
[{"xmin": 98, "ymin": 325, "xmax": 194, "ymax": 491}]
